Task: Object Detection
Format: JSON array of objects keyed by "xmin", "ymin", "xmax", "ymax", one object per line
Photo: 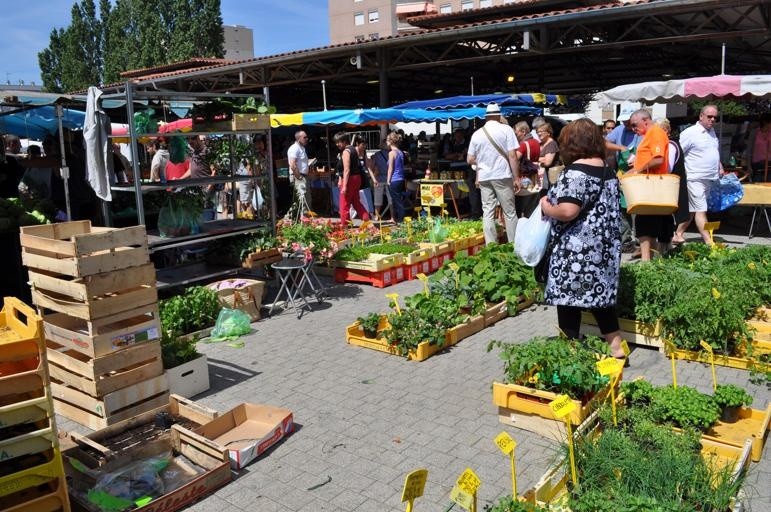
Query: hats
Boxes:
[{"xmin": 484, "ymin": 104, "xmax": 501, "ymax": 116}]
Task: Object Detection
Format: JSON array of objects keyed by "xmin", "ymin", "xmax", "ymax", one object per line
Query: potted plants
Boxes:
[
  {"xmin": 573, "ymin": 240, "xmax": 771, "ymax": 373},
  {"xmin": 457, "ymin": 332, "xmax": 771, "ymax": 511},
  {"xmin": 131, "ymin": 96, "xmax": 550, "ymax": 361}
]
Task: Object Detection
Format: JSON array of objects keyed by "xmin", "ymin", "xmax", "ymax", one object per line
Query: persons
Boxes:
[
  {"xmin": 398, "ymin": 129, "xmax": 469, "ymax": 160},
  {"xmin": 333, "ymin": 132, "xmax": 406, "ymax": 226},
  {"xmin": 1, "ymin": 127, "xmax": 125, "ymax": 223},
  {"xmin": 466, "ymin": 103, "xmax": 560, "ymax": 248},
  {"xmin": 287, "ymin": 130, "xmax": 312, "ymax": 213},
  {"xmin": 734, "ymin": 111, "xmax": 771, "ymax": 183},
  {"xmin": 146, "ymin": 135, "xmax": 269, "ymax": 216},
  {"xmin": 541, "ymin": 118, "xmax": 627, "ymax": 359},
  {"xmin": 602, "ymin": 103, "xmax": 721, "ymax": 262}
]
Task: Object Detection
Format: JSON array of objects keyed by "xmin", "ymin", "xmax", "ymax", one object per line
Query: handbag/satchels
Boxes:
[
  {"xmin": 548, "ymin": 165, "xmax": 565, "ymax": 184},
  {"xmin": 621, "ymin": 163, "xmax": 681, "ymax": 215},
  {"xmin": 534, "ymin": 244, "xmax": 554, "ymax": 283},
  {"xmin": 360, "ymin": 173, "xmax": 370, "ymax": 189},
  {"xmin": 204, "ymin": 279, "xmax": 265, "ymax": 323},
  {"xmin": 708, "ymin": 173, "xmax": 743, "ymax": 213},
  {"xmin": 515, "ymin": 141, "xmax": 545, "ymax": 196}
]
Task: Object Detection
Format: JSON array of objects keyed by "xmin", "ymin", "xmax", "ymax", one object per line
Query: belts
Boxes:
[{"xmin": 291, "ymin": 173, "xmax": 305, "ymax": 176}]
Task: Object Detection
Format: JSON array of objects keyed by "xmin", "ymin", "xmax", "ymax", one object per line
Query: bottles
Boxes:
[{"xmin": 729, "ymin": 155, "xmax": 736, "ymax": 171}]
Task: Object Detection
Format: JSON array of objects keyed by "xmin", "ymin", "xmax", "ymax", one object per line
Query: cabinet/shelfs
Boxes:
[{"xmin": 98, "ymin": 84, "xmax": 275, "ymax": 286}]
